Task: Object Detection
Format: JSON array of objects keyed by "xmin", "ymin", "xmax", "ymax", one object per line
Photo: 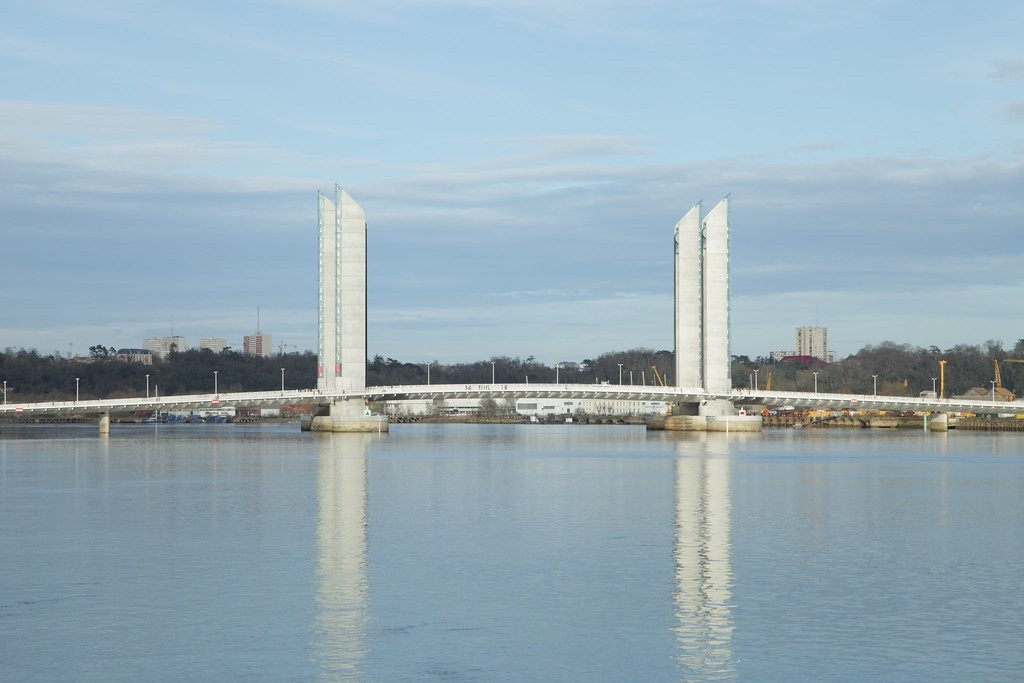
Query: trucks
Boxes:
[{"xmin": 770, "ymin": 406, "xmax": 795, "ymax": 416}]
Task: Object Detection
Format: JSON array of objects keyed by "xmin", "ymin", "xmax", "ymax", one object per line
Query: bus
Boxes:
[{"xmin": 196, "ymin": 407, "xmax": 236, "ymax": 418}]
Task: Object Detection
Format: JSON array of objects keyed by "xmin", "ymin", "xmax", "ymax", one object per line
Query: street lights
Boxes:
[
  {"xmin": 618, "ymin": 363, "xmax": 624, "ymax": 385},
  {"xmin": 990, "ymin": 380, "xmax": 997, "ymax": 408},
  {"xmin": 554, "ymin": 361, "xmax": 561, "ymax": 384},
  {"xmin": 491, "ymin": 361, "xmax": 496, "ymax": 384},
  {"xmin": 146, "ymin": 374, "xmax": 150, "ymax": 398},
  {"xmin": 2, "ymin": 380, "xmax": 8, "ymax": 404},
  {"xmin": 753, "ymin": 370, "xmax": 759, "ymax": 391},
  {"xmin": 280, "ymin": 368, "xmax": 286, "ymax": 390},
  {"xmin": 75, "ymin": 378, "xmax": 79, "ymax": 401},
  {"xmin": 871, "ymin": 375, "xmax": 878, "ymax": 403},
  {"xmin": 214, "ymin": 370, "xmax": 219, "ymax": 393},
  {"xmin": 930, "ymin": 377, "xmax": 937, "ymax": 398},
  {"xmin": 813, "ymin": 372, "xmax": 820, "ymax": 393},
  {"xmin": 426, "ymin": 362, "xmax": 431, "ymax": 385}
]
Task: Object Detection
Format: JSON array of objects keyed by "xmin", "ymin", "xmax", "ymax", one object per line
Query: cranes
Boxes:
[{"xmin": 651, "ymin": 364, "xmax": 673, "ymax": 416}]
